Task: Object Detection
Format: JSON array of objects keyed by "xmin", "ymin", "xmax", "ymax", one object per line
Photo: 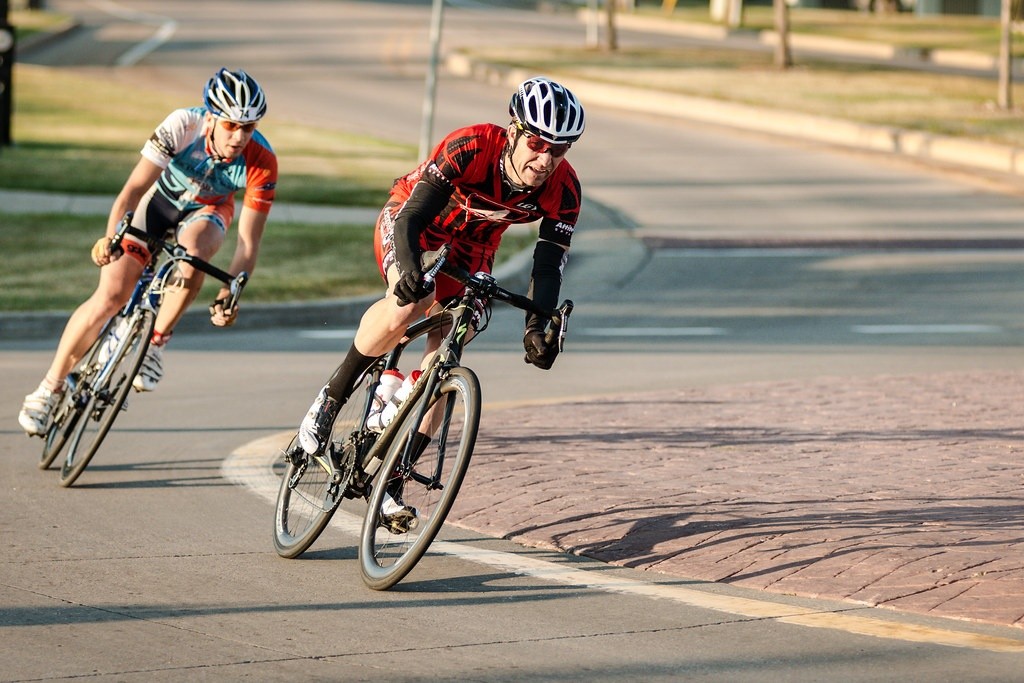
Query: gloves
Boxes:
[
  {"xmin": 524, "ymin": 331, "xmax": 560, "ymax": 370},
  {"xmin": 393, "ymin": 269, "xmax": 437, "ymax": 303}
]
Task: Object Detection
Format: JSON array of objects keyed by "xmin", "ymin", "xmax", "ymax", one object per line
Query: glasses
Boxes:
[
  {"xmin": 212, "ymin": 114, "xmax": 256, "ymax": 133},
  {"xmin": 522, "ymin": 130, "xmax": 569, "ymax": 157}
]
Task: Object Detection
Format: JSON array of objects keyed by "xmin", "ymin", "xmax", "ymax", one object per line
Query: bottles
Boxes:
[
  {"xmin": 108, "ymin": 317, "xmax": 129, "ymax": 353},
  {"xmin": 98, "ymin": 332, "xmax": 112, "ymax": 364},
  {"xmin": 379, "ymin": 368, "xmax": 424, "ymax": 429},
  {"xmin": 366, "ymin": 366, "xmax": 405, "ymax": 431}
]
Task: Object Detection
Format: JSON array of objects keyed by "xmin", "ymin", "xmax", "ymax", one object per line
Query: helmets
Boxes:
[
  {"xmin": 203, "ymin": 68, "xmax": 267, "ymax": 123},
  {"xmin": 508, "ymin": 78, "xmax": 586, "ymax": 144}
]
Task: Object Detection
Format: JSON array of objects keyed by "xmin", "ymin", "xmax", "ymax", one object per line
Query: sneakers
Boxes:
[
  {"xmin": 300, "ymin": 383, "xmax": 349, "ymax": 457},
  {"xmin": 380, "ymin": 482, "xmax": 420, "ymax": 533},
  {"xmin": 18, "ymin": 385, "xmax": 63, "ymax": 435},
  {"xmin": 132, "ymin": 342, "xmax": 165, "ymax": 391}
]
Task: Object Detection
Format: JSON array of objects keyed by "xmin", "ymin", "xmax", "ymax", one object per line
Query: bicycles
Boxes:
[
  {"xmin": 270, "ymin": 245, "xmax": 573, "ymax": 595},
  {"xmin": 26, "ymin": 209, "xmax": 249, "ymax": 488}
]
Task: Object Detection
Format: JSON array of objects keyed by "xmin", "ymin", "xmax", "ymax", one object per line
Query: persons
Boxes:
[
  {"xmin": 17, "ymin": 69, "xmax": 280, "ymax": 438},
  {"xmin": 299, "ymin": 77, "xmax": 588, "ymax": 523}
]
